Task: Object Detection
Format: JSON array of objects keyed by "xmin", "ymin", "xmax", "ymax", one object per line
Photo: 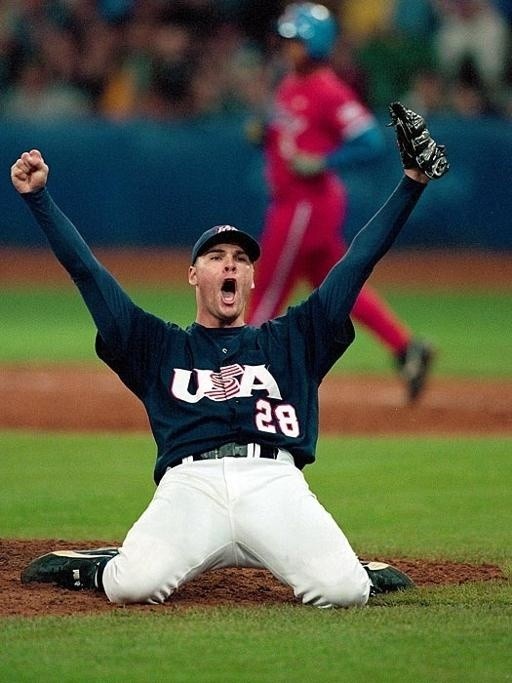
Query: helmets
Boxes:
[{"xmin": 276, "ymin": 2, "xmax": 336, "ymax": 57}]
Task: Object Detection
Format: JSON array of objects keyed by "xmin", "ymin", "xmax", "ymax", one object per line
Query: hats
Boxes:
[{"xmin": 193, "ymin": 224, "xmax": 260, "ymax": 264}]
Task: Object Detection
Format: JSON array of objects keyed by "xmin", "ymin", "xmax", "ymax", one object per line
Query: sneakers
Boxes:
[
  {"xmin": 360, "ymin": 560, "xmax": 417, "ymax": 596},
  {"xmin": 20, "ymin": 546, "xmax": 122, "ymax": 589},
  {"xmin": 398, "ymin": 338, "xmax": 432, "ymax": 404}
]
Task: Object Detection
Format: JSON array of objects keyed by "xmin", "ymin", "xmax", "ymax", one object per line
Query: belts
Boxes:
[{"xmin": 168, "ymin": 442, "xmax": 279, "ymax": 467}]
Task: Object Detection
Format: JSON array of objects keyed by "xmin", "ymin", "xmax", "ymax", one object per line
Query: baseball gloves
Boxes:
[{"xmin": 388, "ymin": 101, "xmax": 450, "ymax": 180}]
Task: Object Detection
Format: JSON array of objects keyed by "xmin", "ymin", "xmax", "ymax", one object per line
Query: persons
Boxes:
[
  {"xmin": 7, "ymin": 102, "xmax": 452, "ymax": 606},
  {"xmin": 1, "ymin": 2, "xmax": 511, "ymax": 133},
  {"xmin": 238, "ymin": 1, "xmax": 437, "ymax": 407}
]
